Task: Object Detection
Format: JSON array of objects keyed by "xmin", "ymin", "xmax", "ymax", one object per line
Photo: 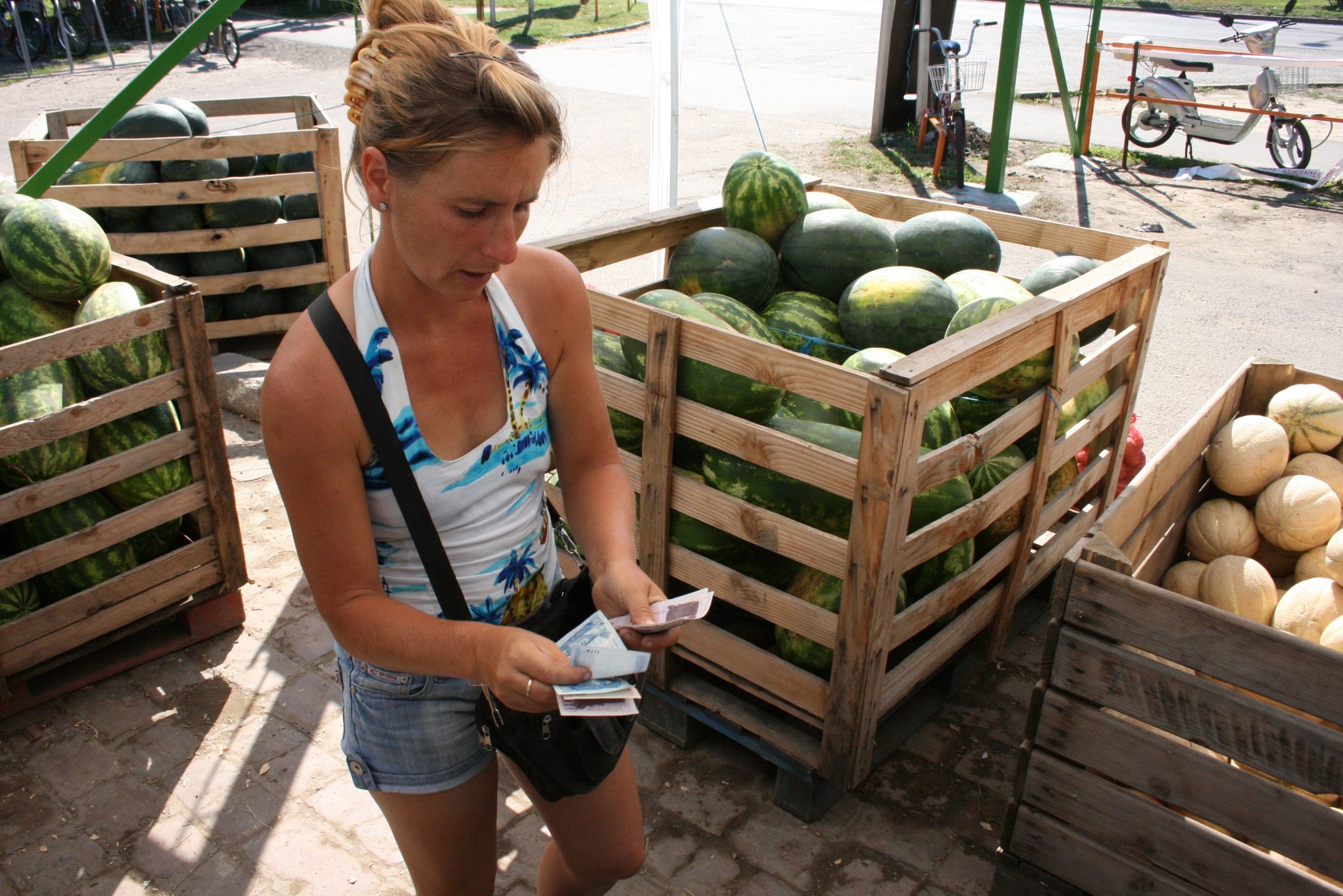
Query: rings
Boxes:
[{"xmin": 527, "ymin": 677, "xmax": 533, "ymax": 698}]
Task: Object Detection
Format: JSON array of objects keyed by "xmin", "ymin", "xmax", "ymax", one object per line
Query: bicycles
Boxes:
[
  {"xmin": 0, "ymin": 0, "xmax": 240, "ymax": 65},
  {"xmin": 913, "ymin": 19, "xmax": 997, "ymax": 188}
]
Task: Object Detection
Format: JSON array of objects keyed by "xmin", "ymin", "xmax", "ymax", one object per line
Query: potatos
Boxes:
[{"xmin": 1074, "ymin": 427, "xmax": 1147, "ymax": 499}]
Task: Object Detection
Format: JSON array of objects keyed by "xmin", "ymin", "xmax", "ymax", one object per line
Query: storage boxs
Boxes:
[
  {"xmin": 5, "ymin": 90, "xmax": 355, "ymax": 358},
  {"xmin": 0, "ymin": 250, "xmax": 253, "ymax": 688},
  {"xmin": 986, "ymin": 355, "xmax": 1343, "ymax": 896},
  {"xmin": 524, "ymin": 166, "xmax": 1166, "ymax": 793}
]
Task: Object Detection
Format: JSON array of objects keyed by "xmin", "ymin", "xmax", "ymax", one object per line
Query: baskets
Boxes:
[
  {"xmin": 1272, "ymin": 66, "xmax": 1308, "ymax": 94},
  {"xmin": 927, "ymin": 61, "xmax": 987, "ymax": 94}
]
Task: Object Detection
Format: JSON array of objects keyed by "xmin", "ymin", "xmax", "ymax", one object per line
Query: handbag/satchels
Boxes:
[{"xmin": 477, "ymin": 565, "xmax": 649, "ymax": 802}]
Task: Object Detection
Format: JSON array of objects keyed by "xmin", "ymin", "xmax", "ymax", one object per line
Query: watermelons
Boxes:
[
  {"xmin": 56, "ymin": 98, "xmax": 323, "ymax": 323},
  {"xmin": 582, "ymin": 152, "xmax": 1117, "ymax": 683},
  {"xmin": 0, "ymin": 192, "xmax": 191, "ymax": 630}
]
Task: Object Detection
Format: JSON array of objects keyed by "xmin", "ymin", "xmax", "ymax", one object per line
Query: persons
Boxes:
[{"xmin": 261, "ymin": 1, "xmax": 683, "ymax": 896}]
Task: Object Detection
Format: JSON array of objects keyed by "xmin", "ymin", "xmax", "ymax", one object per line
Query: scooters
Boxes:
[{"xmin": 1113, "ymin": 0, "xmax": 1311, "ymax": 169}]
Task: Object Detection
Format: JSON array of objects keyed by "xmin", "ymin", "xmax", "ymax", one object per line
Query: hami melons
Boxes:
[
  {"xmin": 1108, "ymin": 646, "xmax": 1343, "ymax": 890},
  {"xmin": 1158, "ymin": 380, "xmax": 1343, "ymax": 657}
]
